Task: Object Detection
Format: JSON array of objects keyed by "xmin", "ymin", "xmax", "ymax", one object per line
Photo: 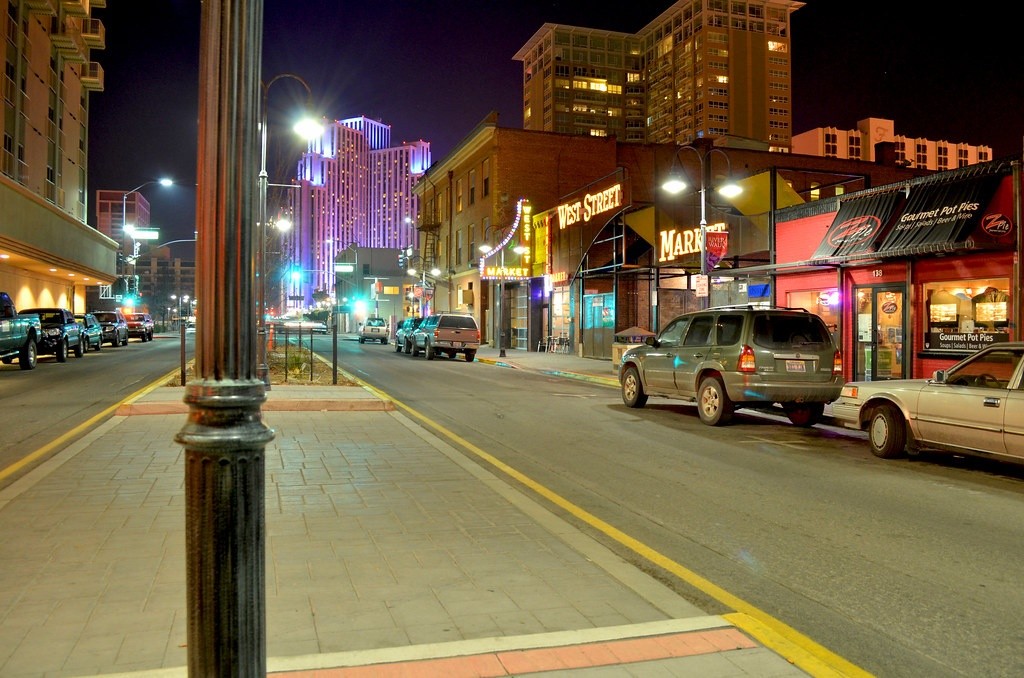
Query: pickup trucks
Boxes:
[{"xmin": 0, "ymin": 291, "xmax": 43, "ymax": 371}]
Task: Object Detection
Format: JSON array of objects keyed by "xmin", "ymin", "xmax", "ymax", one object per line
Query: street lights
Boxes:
[
  {"xmin": 171, "ymin": 294, "xmax": 189, "ymax": 333},
  {"xmin": 124, "ymin": 222, "xmax": 136, "ymax": 280},
  {"xmin": 252, "ymin": 73, "xmax": 327, "ymax": 390},
  {"xmin": 661, "ymin": 145, "xmax": 744, "ymax": 312},
  {"xmin": 408, "ymin": 255, "xmax": 441, "ymax": 322},
  {"xmin": 122, "ymin": 178, "xmax": 173, "ymax": 277},
  {"xmin": 479, "ymin": 223, "xmax": 525, "ymax": 356},
  {"xmin": 167, "ymin": 306, "xmax": 171, "ymax": 328}
]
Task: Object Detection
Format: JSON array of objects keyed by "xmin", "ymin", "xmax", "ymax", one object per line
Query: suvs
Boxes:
[
  {"xmin": 90, "ymin": 310, "xmax": 129, "ymax": 348},
  {"xmin": 617, "ymin": 304, "xmax": 844, "ymax": 426},
  {"xmin": 394, "ymin": 317, "xmax": 424, "ymax": 355},
  {"xmin": 16, "ymin": 307, "xmax": 84, "ymax": 361},
  {"xmin": 410, "ymin": 312, "xmax": 481, "ymax": 362},
  {"xmin": 123, "ymin": 313, "xmax": 155, "ymax": 343},
  {"xmin": 357, "ymin": 317, "xmax": 389, "ymax": 344}
]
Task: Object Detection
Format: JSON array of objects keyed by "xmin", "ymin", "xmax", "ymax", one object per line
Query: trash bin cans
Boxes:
[{"xmin": 611, "ymin": 327, "xmax": 656, "ymax": 375}]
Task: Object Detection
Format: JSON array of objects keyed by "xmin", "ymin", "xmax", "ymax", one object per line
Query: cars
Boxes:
[
  {"xmin": 73, "ymin": 313, "xmax": 105, "ymax": 354},
  {"xmin": 830, "ymin": 340, "xmax": 1024, "ymax": 467}
]
